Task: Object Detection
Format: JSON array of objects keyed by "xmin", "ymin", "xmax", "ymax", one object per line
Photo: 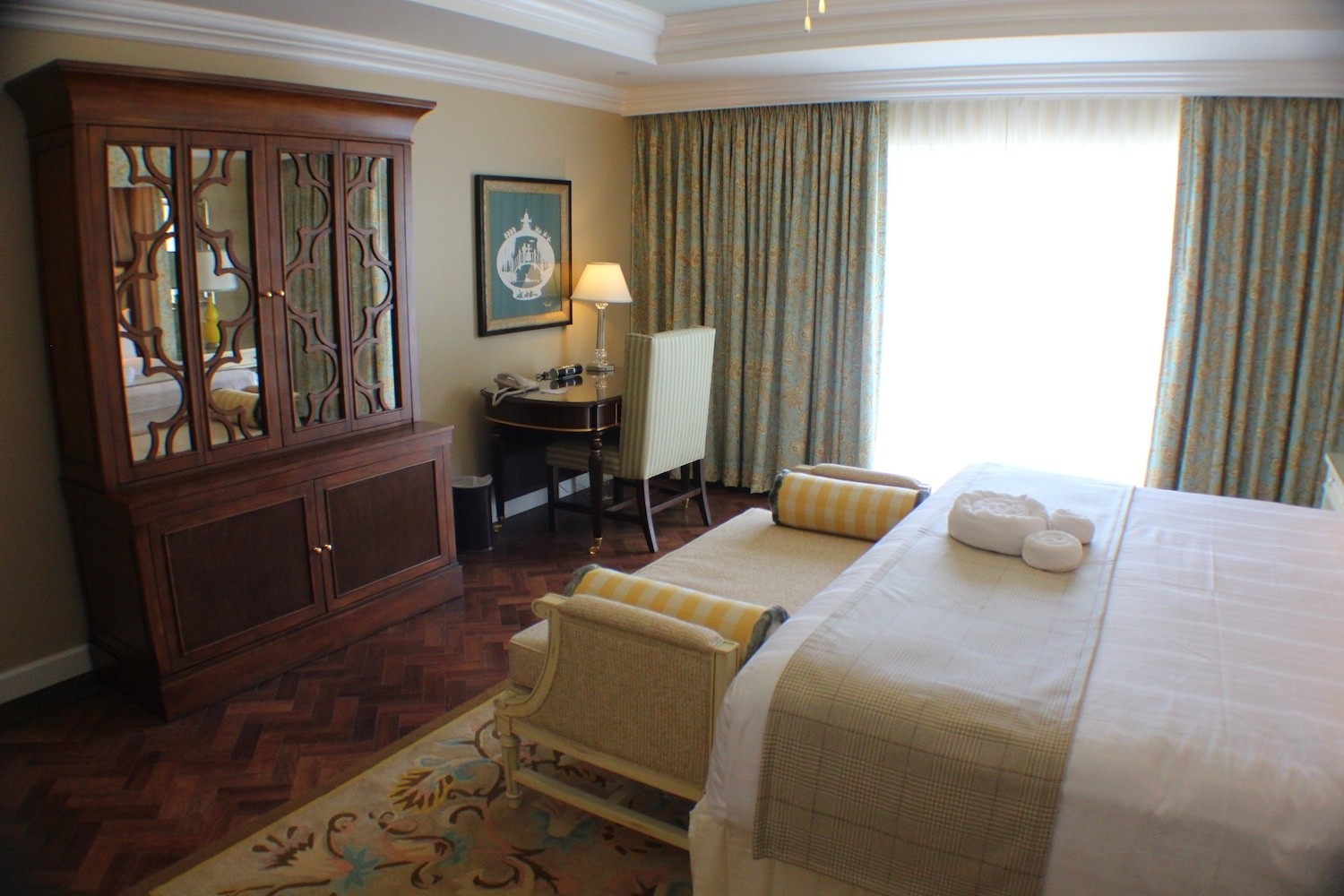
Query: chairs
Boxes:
[{"xmin": 544, "ymin": 325, "xmax": 716, "ymax": 556}]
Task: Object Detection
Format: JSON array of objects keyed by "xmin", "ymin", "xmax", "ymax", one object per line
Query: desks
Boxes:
[{"xmin": 481, "ymin": 365, "xmax": 690, "ymax": 555}]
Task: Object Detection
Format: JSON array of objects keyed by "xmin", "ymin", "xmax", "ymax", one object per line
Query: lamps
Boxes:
[
  {"xmin": 570, "ymin": 262, "xmax": 635, "ymax": 374},
  {"xmin": 195, "ymin": 250, "xmax": 238, "ymax": 352},
  {"xmin": 804, "ymin": 0, "xmax": 825, "ymax": 35}
]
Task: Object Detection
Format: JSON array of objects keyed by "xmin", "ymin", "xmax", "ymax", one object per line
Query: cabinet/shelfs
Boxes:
[{"xmin": 3, "ymin": 58, "xmax": 464, "ymax": 724}]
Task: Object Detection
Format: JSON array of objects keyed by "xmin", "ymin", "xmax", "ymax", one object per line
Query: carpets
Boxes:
[{"xmin": 124, "ymin": 678, "xmax": 703, "ymax": 896}]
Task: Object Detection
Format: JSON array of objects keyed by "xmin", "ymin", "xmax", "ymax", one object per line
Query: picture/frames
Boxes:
[{"xmin": 475, "ymin": 174, "xmax": 573, "ymax": 339}]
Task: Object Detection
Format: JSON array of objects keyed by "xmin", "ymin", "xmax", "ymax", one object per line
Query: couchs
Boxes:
[
  {"xmin": 492, "ymin": 462, "xmax": 929, "ymax": 856},
  {"xmin": 128, "ymin": 385, "xmax": 301, "ymax": 466}
]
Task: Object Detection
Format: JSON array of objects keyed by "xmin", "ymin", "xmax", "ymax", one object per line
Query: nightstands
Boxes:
[{"xmin": 1318, "ymin": 453, "xmax": 1344, "ymax": 513}]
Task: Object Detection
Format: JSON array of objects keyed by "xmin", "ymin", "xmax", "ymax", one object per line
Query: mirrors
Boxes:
[
  {"xmin": 188, "ymin": 146, "xmax": 272, "ymax": 453},
  {"xmin": 341, "ymin": 151, "xmax": 406, "ymax": 425},
  {"xmin": 107, "ymin": 143, "xmax": 197, "ymax": 468},
  {"xmin": 279, "ymin": 146, "xmax": 348, "ymax": 435}
]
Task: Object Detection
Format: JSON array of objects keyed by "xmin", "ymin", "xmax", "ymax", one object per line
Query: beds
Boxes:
[
  {"xmin": 682, "ymin": 461, "xmax": 1344, "ymax": 896},
  {"xmin": 119, "ymin": 308, "xmax": 260, "ymax": 441}
]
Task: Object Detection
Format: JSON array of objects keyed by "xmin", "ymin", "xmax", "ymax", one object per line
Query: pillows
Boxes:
[
  {"xmin": 209, "ymin": 388, "xmax": 261, "ymax": 429},
  {"xmin": 560, "ymin": 563, "xmax": 790, "ymax": 667},
  {"xmin": 766, "ymin": 467, "xmax": 929, "ymax": 546}
]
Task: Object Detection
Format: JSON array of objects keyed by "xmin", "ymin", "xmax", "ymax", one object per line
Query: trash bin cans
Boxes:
[{"xmin": 451, "ymin": 476, "xmax": 493, "ymax": 554}]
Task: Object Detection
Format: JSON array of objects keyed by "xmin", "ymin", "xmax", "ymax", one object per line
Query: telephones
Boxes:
[{"xmin": 494, "ymin": 372, "xmax": 540, "ymax": 392}]
